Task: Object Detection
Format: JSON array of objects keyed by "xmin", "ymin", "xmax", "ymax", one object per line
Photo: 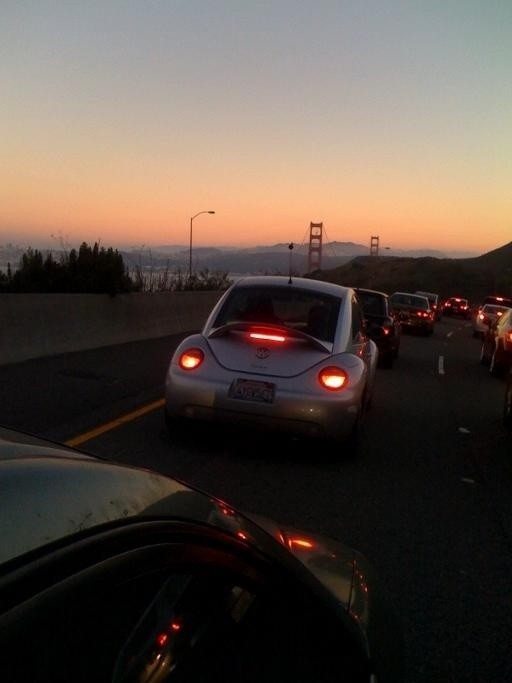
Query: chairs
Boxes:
[
  {"xmin": 246, "ymin": 302, "xmax": 273, "ymax": 314},
  {"xmin": 308, "ymin": 304, "xmax": 330, "ymax": 330},
  {"xmin": 362, "ymin": 304, "xmax": 376, "ymax": 313}
]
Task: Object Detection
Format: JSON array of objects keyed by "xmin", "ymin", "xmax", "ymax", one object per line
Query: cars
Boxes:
[
  {"xmin": 0, "ymin": 419, "xmax": 382, "ymax": 682},
  {"xmin": 161, "ymin": 241, "xmax": 383, "ymax": 445},
  {"xmin": 348, "ymin": 286, "xmax": 512, "ymax": 375}
]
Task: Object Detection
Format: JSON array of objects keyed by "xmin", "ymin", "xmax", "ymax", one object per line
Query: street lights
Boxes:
[
  {"xmin": 189, "ymin": 209, "xmax": 217, "ymax": 274},
  {"xmin": 374, "ymin": 245, "xmax": 392, "ymax": 261}
]
